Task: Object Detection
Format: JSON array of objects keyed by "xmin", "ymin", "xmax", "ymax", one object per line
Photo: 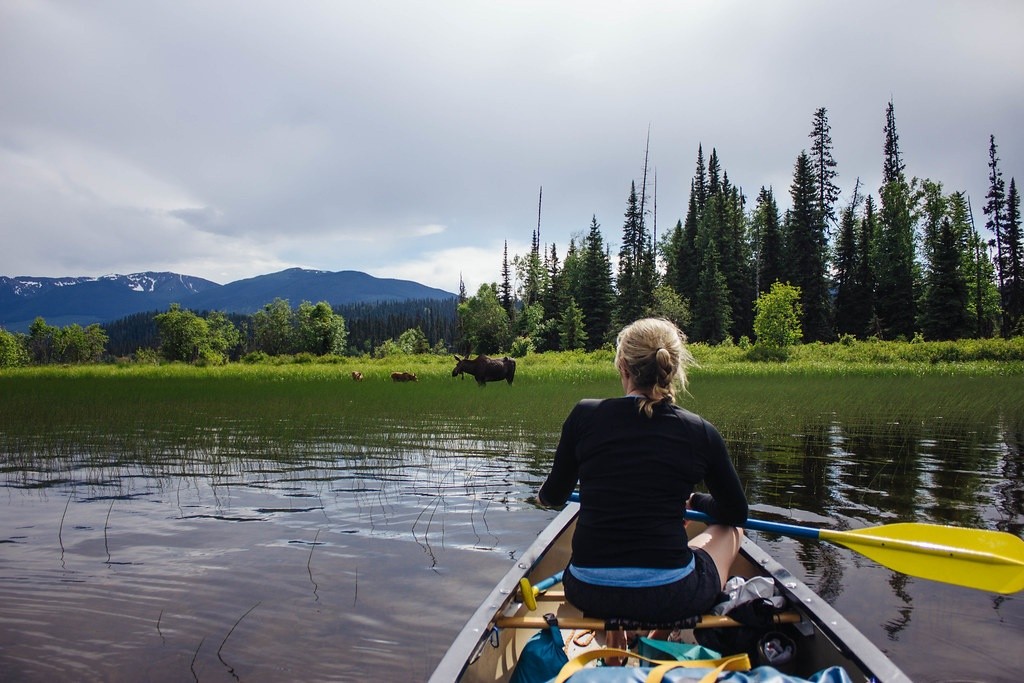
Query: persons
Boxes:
[{"xmin": 538, "ymin": 319, "xmax": 749, "ymax": 666}]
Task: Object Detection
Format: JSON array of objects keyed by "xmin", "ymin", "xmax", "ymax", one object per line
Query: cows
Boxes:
[
  {"xmin": 352, "ymin": 371, "xmax": 363, "ymax": 382},
  {"xmin": 391, "ymin": 371, "xmax": 418, "ymax": 383},
  {"xmin": 451, "ymin": 352, "xmax": 516, "ymax": 388}
]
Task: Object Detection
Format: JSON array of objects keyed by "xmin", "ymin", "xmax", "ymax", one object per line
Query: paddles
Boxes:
[
  {"xmin": 519, "ymin": 566, "xmax": 567, "ymax": 613},
  {"xmin": 565, "ymin": 489, "xmax": 1023, "ymax": 597}
]
